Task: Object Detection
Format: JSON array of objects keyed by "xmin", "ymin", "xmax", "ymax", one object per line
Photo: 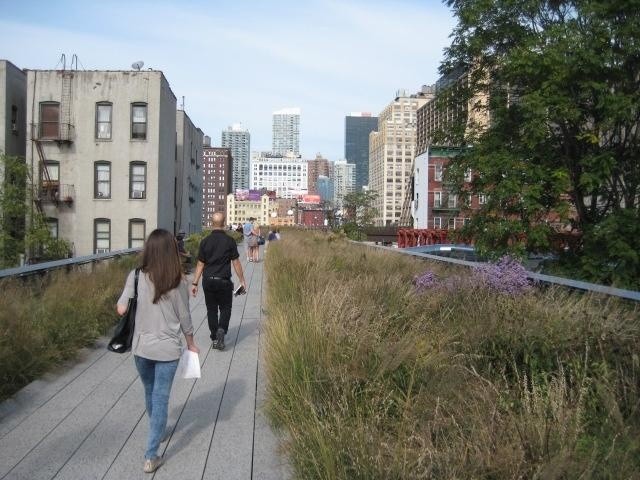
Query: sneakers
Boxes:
[
  {"xmin": 216, "ymin": 330, "xmax": 225, "ymax": 350},
  {"xmin": 144, "ymin": 456, "xmax": 164, "ymax": 473},
  {"xmin": 213, "ymin": 342, "xmax": 218, "ymax": 349}
]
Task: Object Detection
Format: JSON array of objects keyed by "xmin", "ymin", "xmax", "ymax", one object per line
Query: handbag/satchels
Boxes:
[
  {"xmin": 107, "ymin": 267, "xmax": 140, "ymax": 353},
  {"xmin": 257, "ymin": 234, "xmax": 265, "ymax": 245}
]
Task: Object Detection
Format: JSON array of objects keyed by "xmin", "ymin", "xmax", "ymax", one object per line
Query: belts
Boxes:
[{"xmin": 207, "ymin": 276, "xmax": 225, "ymax": 280}]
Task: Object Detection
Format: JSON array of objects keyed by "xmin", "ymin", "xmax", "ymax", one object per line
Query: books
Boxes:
[{"xmin": 234, "ymin": 285, "xmax": 244, "ymax": 297}]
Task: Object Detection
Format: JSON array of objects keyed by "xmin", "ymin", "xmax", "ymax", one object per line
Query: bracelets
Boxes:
[{"xmin": 193, "ymin": 282, "xmax": 198, "ymax": 286}]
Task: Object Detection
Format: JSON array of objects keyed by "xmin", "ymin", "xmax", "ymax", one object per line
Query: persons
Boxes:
[
  {"xmin": 175, "ymin": 228, "xmax": 193, "ymax": 274},
  {"xmin": 190, "ymin": 211, "xmax": 246, "ymax": 351},
  {"xmin": 229, "ymin": 216, "xmax": 281, "ymax": 263},
  {"xmin": 116, "ymin": 228, "xmax": 201, "ymax": 474}
]
